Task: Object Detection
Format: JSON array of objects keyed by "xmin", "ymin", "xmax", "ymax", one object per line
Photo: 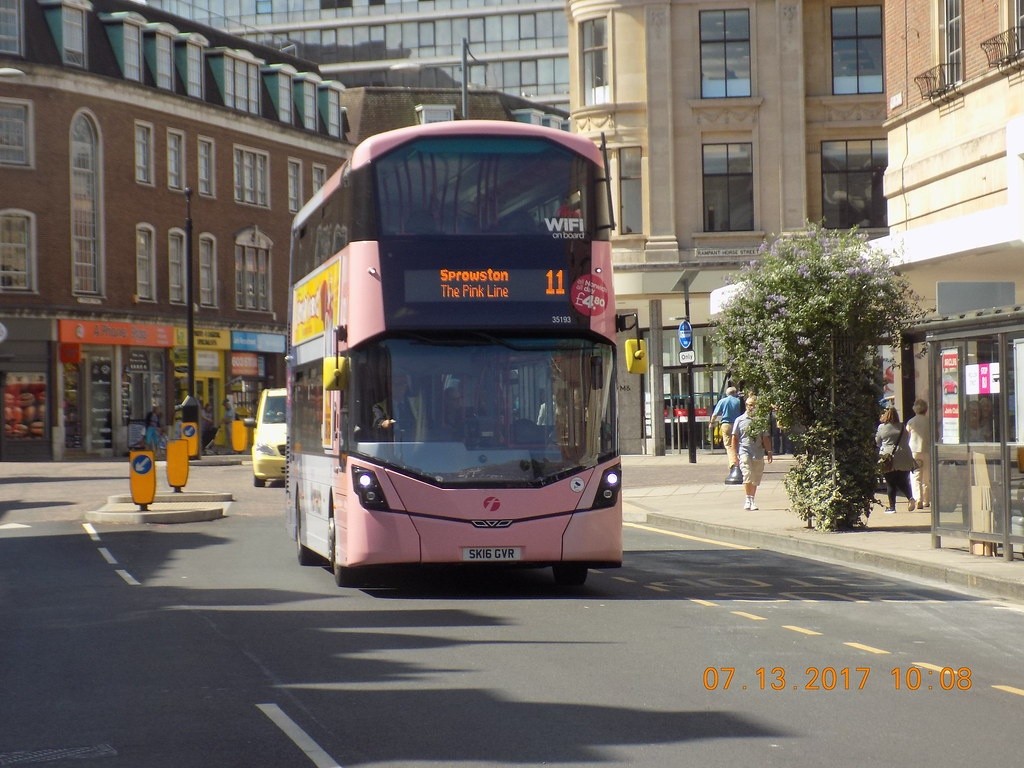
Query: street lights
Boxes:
[{"xmin": 670, "ymin": 266, "xmax": 703, "ymax": 463}]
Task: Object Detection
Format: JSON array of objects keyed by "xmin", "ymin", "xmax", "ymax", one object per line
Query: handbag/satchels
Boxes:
[{"xmin": 877, "ymin": 454, "xmax": 894, "ymax": 473}]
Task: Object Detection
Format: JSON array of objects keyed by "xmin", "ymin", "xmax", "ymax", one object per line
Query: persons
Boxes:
[
  {"xmin": 367, "ymin": 369, "xmax": 430, "ymax": 444},
  {"xmin": 707, "ymin": 387, "xmax": 740, "ymax": 468},
  {"xmin": 733, "ymin": 398, "xmax": 773, "ymax": 511},
  {"xmin": 906, "ymin": 399, "xmax": 932, "ymax": 509},
  {"xmin": 199, "ymin": 400, "xmax": 238, "ymax": 453},
  {"xmin": 875, "ymin": 408, "xmax": 916, "ymax": 513}
]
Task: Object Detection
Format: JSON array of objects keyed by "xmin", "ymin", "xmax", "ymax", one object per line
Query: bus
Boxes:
[{"xmin": 285, "ymin": 104, "xmax": 647, "ymax": 589}]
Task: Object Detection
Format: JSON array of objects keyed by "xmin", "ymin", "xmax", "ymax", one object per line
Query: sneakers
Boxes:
[
  {"xmin": 884, "ymin": 497, "xmax": 930, "ymax": 514},
  {"xmin": 744, "ymin": 495, "xmax": 758, "ymax": 510}
]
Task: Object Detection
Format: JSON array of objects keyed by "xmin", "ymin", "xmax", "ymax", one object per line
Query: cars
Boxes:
[
  {"xmin": 943, "ymin": 374, "xmax": 958, "ymax": 394},
  {"xmin": 243, "ymin": 389, "xmax": 293, "ymax": 488}
]
